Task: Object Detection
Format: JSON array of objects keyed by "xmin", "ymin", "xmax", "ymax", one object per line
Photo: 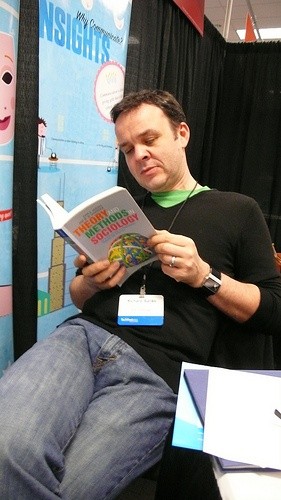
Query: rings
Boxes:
[{"xmin": 169, "ymin": 255, "xmax": 175, "ymax": 267}]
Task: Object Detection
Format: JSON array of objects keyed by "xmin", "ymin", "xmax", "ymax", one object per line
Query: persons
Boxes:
[{"xmin": 0, "ymin": 89, "xmax": 280, "ymax": 500}]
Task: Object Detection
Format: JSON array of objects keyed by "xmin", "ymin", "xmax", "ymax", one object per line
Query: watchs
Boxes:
[{"xmin": 195, "ymin": 267, "xmax": 221, "ymax": 300}]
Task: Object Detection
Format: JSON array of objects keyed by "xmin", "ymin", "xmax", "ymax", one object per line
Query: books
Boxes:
[
  {"xmin": 170, "ymin": 360, "xmax": 280, "ymax": 472},
  {"xmin": 35, "ymin": 186, "xmax": 159, "ymax": 288}
]
